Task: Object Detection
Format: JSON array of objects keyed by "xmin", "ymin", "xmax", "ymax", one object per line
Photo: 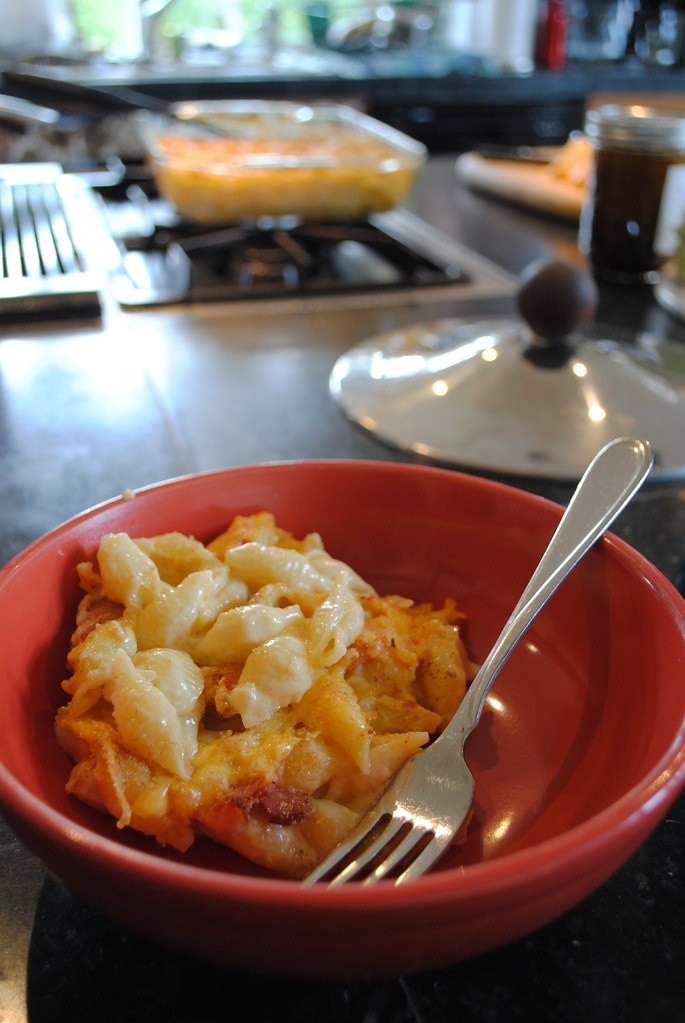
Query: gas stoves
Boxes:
[{"xmin": 78, "ymin": 186, "xmax": 522, "ymax": 311}]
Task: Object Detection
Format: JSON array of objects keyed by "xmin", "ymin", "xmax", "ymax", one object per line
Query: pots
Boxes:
[{"xmin": 0, "ymin": 59, "xmax": 427, "ymax": 222}]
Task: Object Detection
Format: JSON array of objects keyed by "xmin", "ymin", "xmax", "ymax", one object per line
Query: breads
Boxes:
[{"xmin": 457, "ymin": 151, "xmax": 589, "ymax": 217}]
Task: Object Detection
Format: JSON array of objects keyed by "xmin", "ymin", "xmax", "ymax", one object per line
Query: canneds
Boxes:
[{"xmin": 577, "ymin": 102, "xmax": 685, "ymax": 267}]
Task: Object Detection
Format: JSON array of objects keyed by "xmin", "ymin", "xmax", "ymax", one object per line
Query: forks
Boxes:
[{"xmin": 287, "ymin": 438, "xmax": 654, "ymax": 897}]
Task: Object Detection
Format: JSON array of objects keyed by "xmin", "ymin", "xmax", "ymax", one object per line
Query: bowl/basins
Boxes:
[{"xmin": 1, "ymin": 457, "xmax": 685, "ymax": 980}]
